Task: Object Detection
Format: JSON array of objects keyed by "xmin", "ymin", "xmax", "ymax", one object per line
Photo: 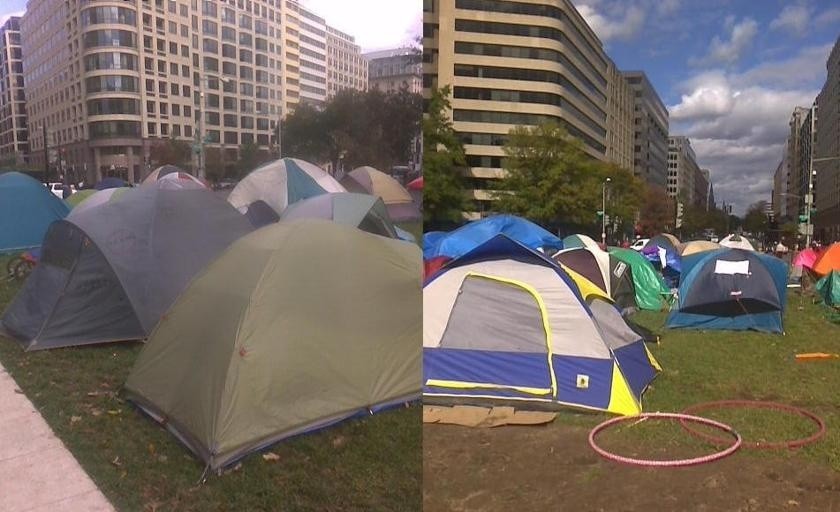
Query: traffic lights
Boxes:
[
  {"xmin": 676, "ymin": 202, "xmax": 684, "ymax": 219},
  {"xmin": 60, "ymin": 148, "xmax": 66, "ymax": 152},
  {"xmin": 676, "ymin": 218, "xmax": 682, "ymax": 230}
]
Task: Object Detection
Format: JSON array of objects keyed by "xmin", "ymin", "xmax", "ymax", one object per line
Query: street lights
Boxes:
[
  {"xmin": 196, "ymin": 76, "xmax": 230, "ymax": 180},
  {"xmin": 255, "ymin": 110, "xmax": 282, "ymax": 160},
  {"xmin": 774, "ymin": 192, "xmax": 810, "ymax": 249},
  {"xmin": 600, "ymin": 177, "xmax": 612, "ymax": 244}
]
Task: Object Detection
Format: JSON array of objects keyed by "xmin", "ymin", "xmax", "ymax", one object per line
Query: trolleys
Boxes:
[{"xmin": 1, "ymin": 253, "xmax": 37, "ymax": 284}]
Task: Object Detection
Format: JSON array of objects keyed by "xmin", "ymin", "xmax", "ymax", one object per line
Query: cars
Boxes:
[
  {"xmin": 216, "ymin": 177, "xmax": 238, "ymax": 189},
  {"xmin": 703, "ymin": 227, "xmax": 756, "ymax": 247},
  {"xmin": 387, "ymin": 165, "xmax": 410, "ymax": 178},
  {"xmin": 40, "ymin": 183, "xmax": 78, "ymax": 198}
]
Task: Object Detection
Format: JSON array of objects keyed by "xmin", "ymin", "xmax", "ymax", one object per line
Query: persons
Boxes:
[{"xmin": 423, "ymin": 233, "xmax": 661, "ymax": 419}]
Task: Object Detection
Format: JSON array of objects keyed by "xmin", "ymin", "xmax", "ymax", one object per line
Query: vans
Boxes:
[{"xmin": 629, "ymin": 238, "xmax": 651, "ymax": 251}]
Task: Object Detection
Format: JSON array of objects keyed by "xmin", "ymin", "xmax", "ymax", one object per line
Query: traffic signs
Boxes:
[
  {"xmin": 798, "ymin": 215, "xmax": 808, "ymax": 221},
  {"xmin": 810, "ymin": 208, "xmax": 818, "ymax": 214}
]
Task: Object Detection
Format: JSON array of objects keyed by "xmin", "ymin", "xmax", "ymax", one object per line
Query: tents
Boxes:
[
  {"xmin": 0, "ymin": 170, "xmax": 75, "ymax": 253},
  {"xmin": 64, "ymin": 156, "xmax": 423, "ymax": 244},
  {"xmin": 119, "ymin": 219, "xmax": 422, "ymax": 472},
  {"xmin": 426, "ymin": 213, "xmax": 840, "ymax": 335},
  {"xmin": 1, "ymin": 186, "xmax": 255, "ymax": 352}
]
